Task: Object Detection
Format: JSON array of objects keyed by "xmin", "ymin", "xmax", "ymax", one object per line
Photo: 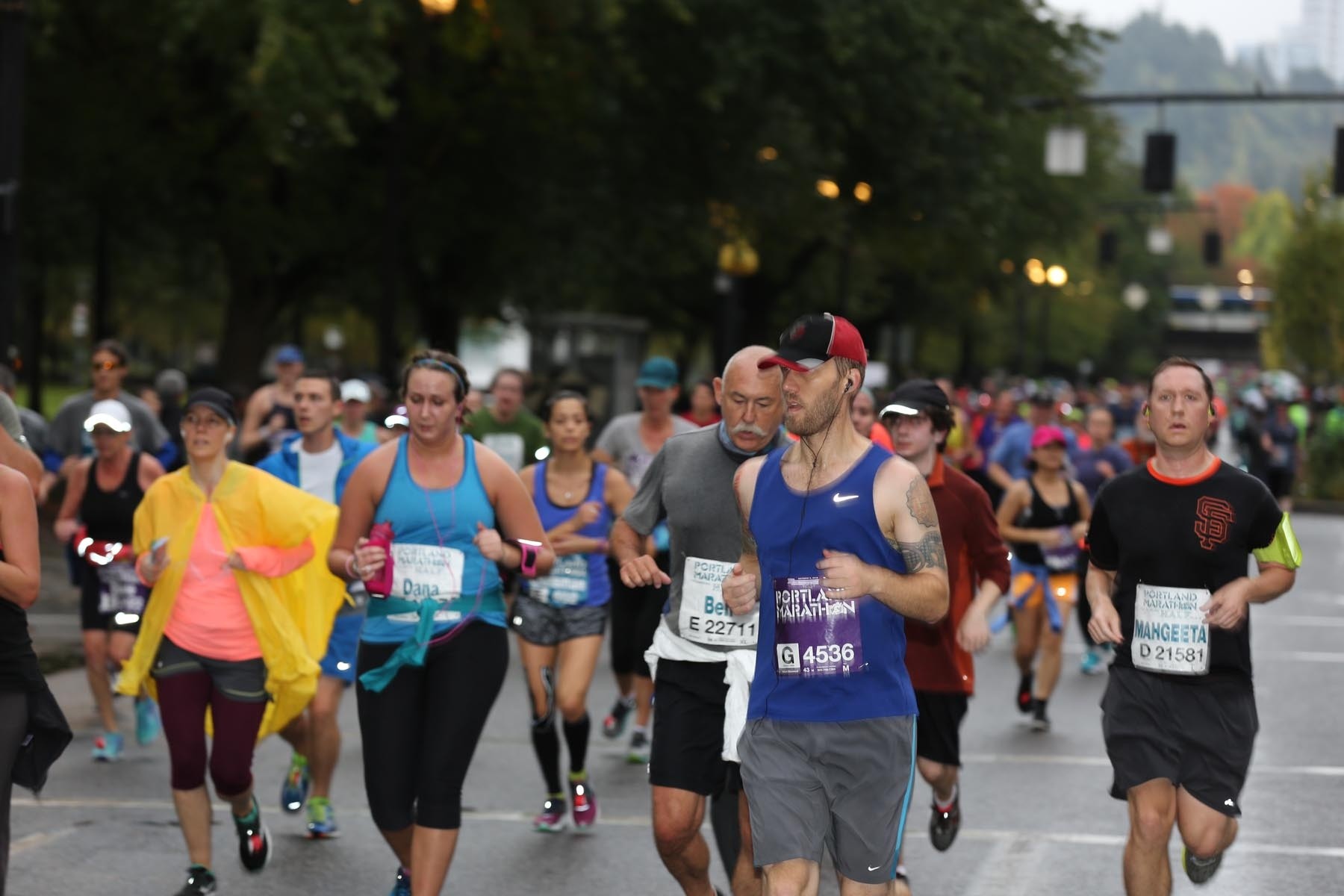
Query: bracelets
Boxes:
[{"xmin": 594, "ymin": 539, "xmax": 607, "ymax": 554}]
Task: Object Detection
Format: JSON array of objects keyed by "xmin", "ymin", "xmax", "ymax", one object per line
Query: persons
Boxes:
[
  {"xmin": 1085, "ymin": 357, "xmax": 1300, "ymax": 896},
  {"xmin": 505, "ymin": 390, "xmax": 656, "ymax": 834},
  {"xmin": 327, "ymin": 351, "xmax": 555, "ymax": 896},
  {"xmin": 0, "ymin": 345, "xmax": 1344, "ymax": 896},
  {"xmin": 720, "ymin": 311, "xmax": 949, "ymax": 896}
]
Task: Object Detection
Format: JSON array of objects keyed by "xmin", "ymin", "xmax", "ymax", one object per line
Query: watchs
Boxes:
[{"xmin": 507, "ymin": 536, "xmax": 542, "ymax": 579}]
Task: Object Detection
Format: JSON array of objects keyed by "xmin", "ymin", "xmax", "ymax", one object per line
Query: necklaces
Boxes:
[{"xmin": 562, "ymin": 492, "xmax": 572, "ymax": 499}]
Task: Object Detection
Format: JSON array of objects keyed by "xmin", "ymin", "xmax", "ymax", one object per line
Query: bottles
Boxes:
[{"xmin": 362, "ymin": 521, "xmax": 395, "ymax": 600}]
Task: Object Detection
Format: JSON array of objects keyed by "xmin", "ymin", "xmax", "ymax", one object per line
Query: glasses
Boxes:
[{"xmin": 94, "ymin": 361, "xmax": 118, "ymax": 371}]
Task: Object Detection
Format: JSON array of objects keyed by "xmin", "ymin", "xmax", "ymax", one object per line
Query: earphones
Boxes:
[{"xmin": 844, "ymin": 380, "xmax": 853, "ymax": 391}]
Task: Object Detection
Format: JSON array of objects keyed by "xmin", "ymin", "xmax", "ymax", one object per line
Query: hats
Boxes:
[
  {"xmin": 1030, "ymin": 425, "xmax": 1067, "ymax": 446},
  {"xmin": 635, "ymin": 356, "xmax": 677, "ymax": 390},
  {"xmin": 83, "ymin": 398, "xmax": 133, "ymax": 433},
  {"xmin": 341, "ymin": 378, "xmax": 373, "ymax": 404},
  {"xmin": 877, "ymin": 379, "xmax": 950, "ymax": 424},
  {"xmin": 181, "ymin": 389, "xmax": 237, "ymax": 425},
  {"xmin": 757, "ymin": 313, "xmax": 868, "ymax": 372},
  {"xmin": 277, "ymin": 346, "xmax": 302, "ymax": 364}
]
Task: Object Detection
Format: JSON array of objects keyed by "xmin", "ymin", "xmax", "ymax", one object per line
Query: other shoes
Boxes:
[
  {"xmin": 1032, "ymin": 713, "xmax": 1048, "ymax": 731},
  {"xmin": 281, "ymin": 753, "xmax": 309, "ymax": 813},
  {"xmin": 569, "ymin": 781, "xmax": 596, "ymax": 829},
  {"xmin": 176, "ymin": 865, "xmax": 217, "ymax": 896},
  {"xmin": 308, "ymin": 796, "xmax": 340, "ymax": 838},
  {"xmin": 604, "ymin": 702, "xmax": 630, "ymax": 737},
  {"xmin": 136, "ymin": 698, "xmax": 160, "ymax": 742},
  {"xmin": 532, "ymin": 797, "xmax": 566, "ymax": 832},
  {"xmin": 392, "ymin": 866, "xmax": 410, "ymax": 896},
  {"xmin": 1183, "ymin": 843, "xmax": 1222, "ymax": 884},
  {"xmin": 929, "ymin": 788, "xmax": 960, "ymax": 851},
  {"xmin": 629, "ymin": 732, "xmax": 647, "ymax": 763},
  {"xmin": 1018, "ymin": 675, "xmax": 1034, "ymax": 711},
  {"xmin": 1081, "ymin": 646, "xmax": 1118, "ymax": 676},
  {"xmin": 90, "ymin": 732, "xmax": 122, "ymax": 761},
  {"xmin": 232, "ymin": 795, "xmax": 273, "ymax": 874}
]
